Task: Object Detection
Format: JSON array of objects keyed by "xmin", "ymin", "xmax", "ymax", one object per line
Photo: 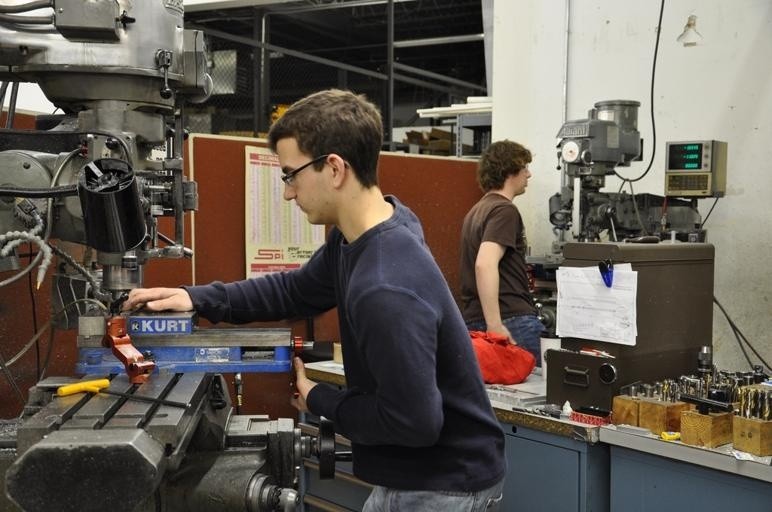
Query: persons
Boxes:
[
  {"xmin": 456, "ymin": 140, "xmax": 546, "ymax": 373},
  {"xmin": 117, "ymin": 86, "xmax": 507, "ymax": 512}
]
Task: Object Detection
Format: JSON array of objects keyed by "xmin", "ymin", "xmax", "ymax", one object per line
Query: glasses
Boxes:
[{"xmin": 281, "ymin": 155, "xmax": 350, "ymax": 186}]
